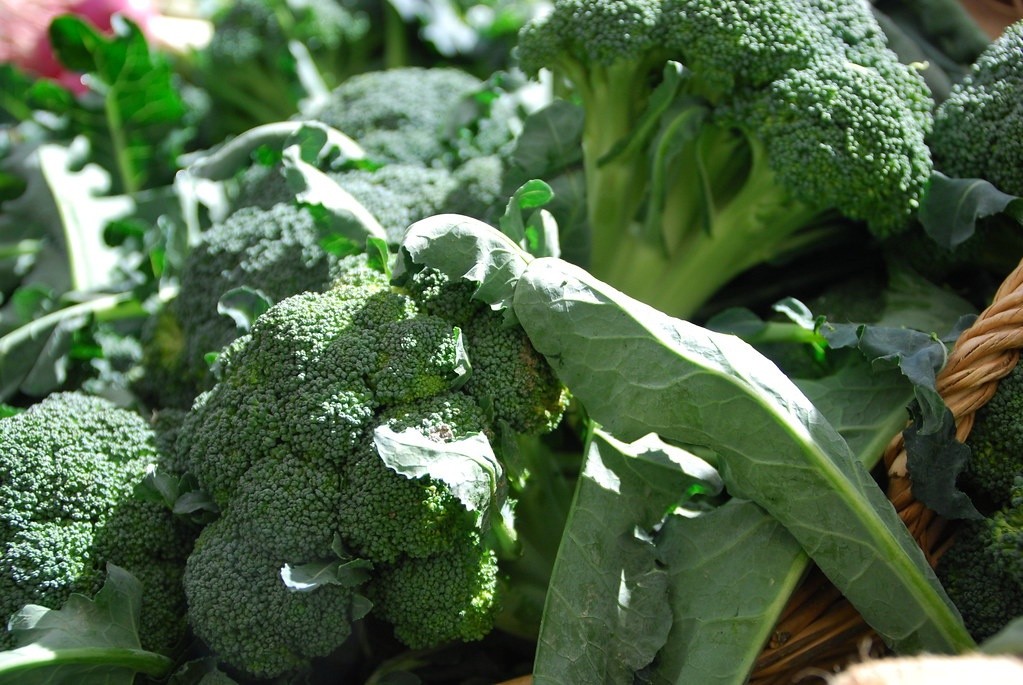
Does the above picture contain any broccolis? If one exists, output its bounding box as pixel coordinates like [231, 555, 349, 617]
[0, 0, 1023, 685]
